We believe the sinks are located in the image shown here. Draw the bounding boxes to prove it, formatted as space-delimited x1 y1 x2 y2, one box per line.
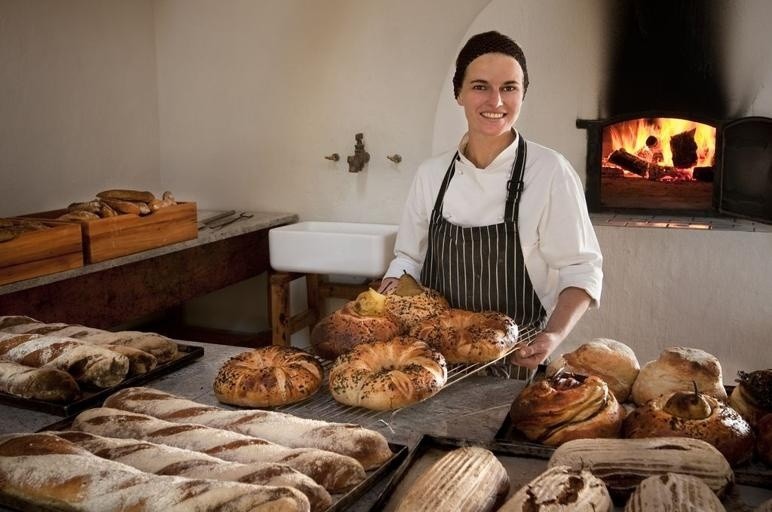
269 220 400 279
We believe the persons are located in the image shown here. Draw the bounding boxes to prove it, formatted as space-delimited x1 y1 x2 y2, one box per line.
378 31 603 382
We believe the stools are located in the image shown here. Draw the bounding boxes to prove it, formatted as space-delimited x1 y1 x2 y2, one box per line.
270 267 379 347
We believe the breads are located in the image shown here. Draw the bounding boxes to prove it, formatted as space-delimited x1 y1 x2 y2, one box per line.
409 308 518 362
547 437 735 499
0 331 129 387
533 338 640 405
510 372 627 445
71 406 366 494
329 336 447 410
624 473 726 512
0 315 178 364
632 345 727 407
620 380 754 470
0 432 311 512
101 343 157 374
0 361 80 401
730 369 772 425
103 386 392 472
396 446 510 512
48 430 333 510
310 269 450 358
497 465 614 512
213 343 324 408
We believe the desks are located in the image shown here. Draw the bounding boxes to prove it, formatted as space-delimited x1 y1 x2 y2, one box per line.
0 324 770 512
2 195 298 326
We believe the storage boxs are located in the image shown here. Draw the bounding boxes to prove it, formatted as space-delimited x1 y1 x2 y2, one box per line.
1 217 85 287
21 182 196 266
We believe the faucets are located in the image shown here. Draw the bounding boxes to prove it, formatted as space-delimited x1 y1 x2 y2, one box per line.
347 132 371 172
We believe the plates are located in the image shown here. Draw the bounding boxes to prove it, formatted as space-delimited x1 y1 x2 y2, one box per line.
1 343 206 417
370 434 771 511
36 405 410 512
494 365 771 488
